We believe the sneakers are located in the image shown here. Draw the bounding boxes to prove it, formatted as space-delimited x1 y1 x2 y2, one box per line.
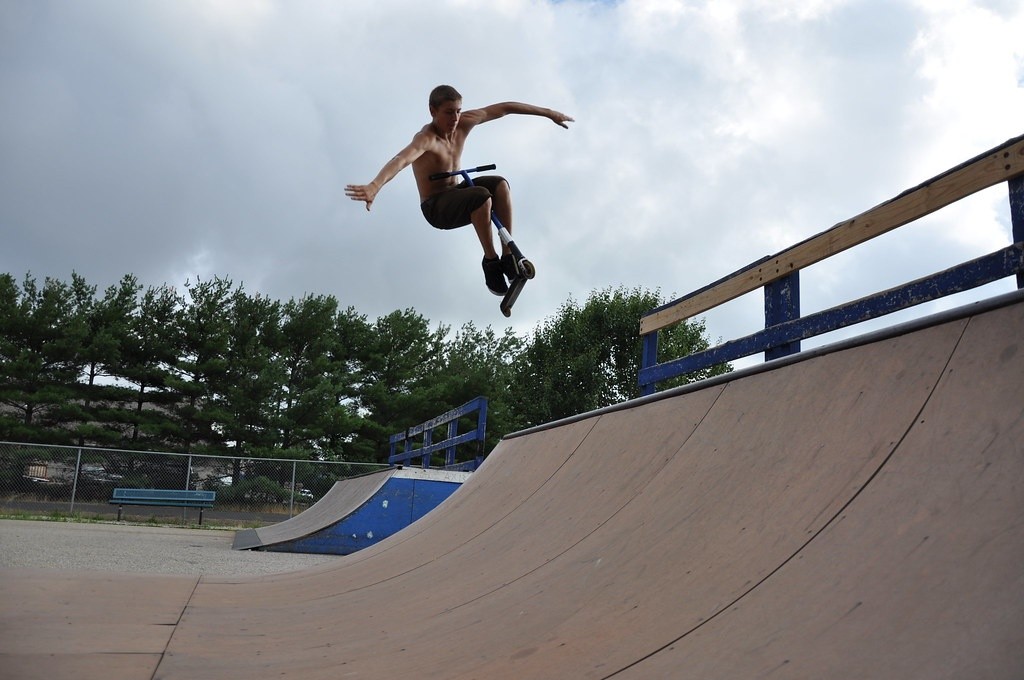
482 257 509 296
500 257 518 283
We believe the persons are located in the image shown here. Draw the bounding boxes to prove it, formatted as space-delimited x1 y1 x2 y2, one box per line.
344 85 575 296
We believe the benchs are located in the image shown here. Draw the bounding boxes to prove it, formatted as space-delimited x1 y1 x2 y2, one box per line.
109 488 216 525
